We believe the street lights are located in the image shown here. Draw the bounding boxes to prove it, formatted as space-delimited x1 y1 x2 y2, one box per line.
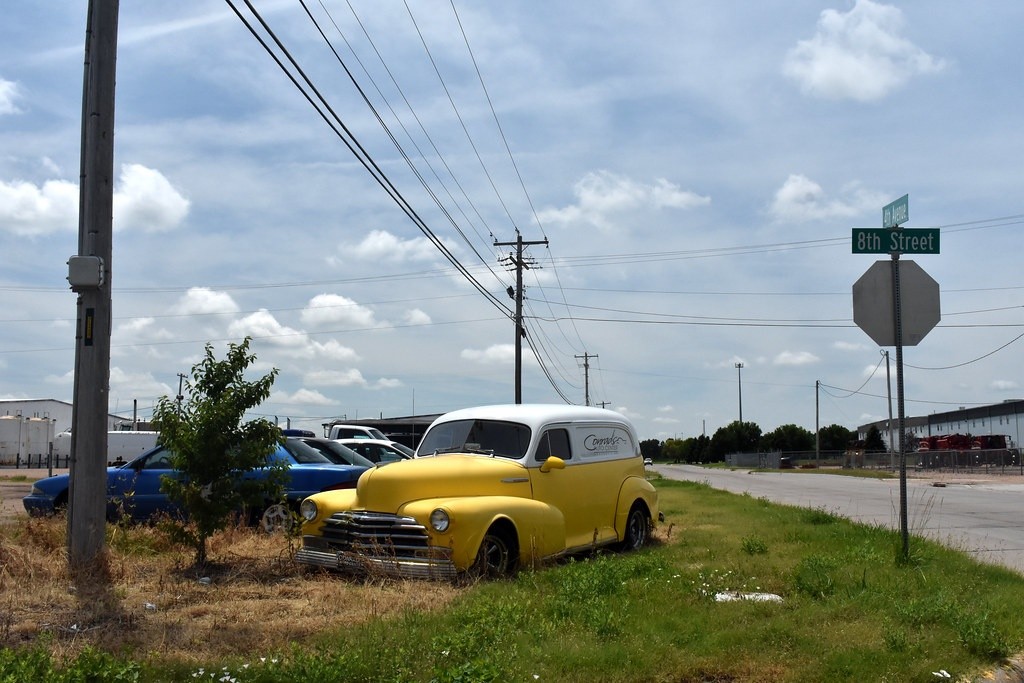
734 361 744 423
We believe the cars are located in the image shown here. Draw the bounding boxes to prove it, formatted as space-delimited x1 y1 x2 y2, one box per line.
766 455 792 468
644 458 653 466
293 404 659 585
21 425 415 537
